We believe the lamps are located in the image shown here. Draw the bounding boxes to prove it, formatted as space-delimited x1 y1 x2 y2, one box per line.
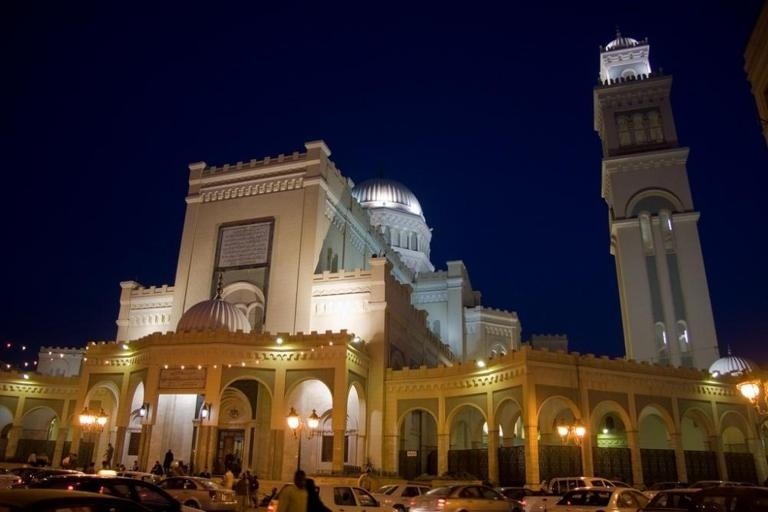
201 402 211 421
139 402 150 419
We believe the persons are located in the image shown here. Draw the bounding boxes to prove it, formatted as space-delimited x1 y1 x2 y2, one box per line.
28 450 37 467
358 468 372 491
391 503 407 512
150 449 329 511
62 442 140 473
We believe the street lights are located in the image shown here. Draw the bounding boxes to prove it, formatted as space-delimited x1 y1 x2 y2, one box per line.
139 401 151 471
201 401 213 466
735 368 768 428
555 417 588 477
78 407 109 465
285 407 321 471
